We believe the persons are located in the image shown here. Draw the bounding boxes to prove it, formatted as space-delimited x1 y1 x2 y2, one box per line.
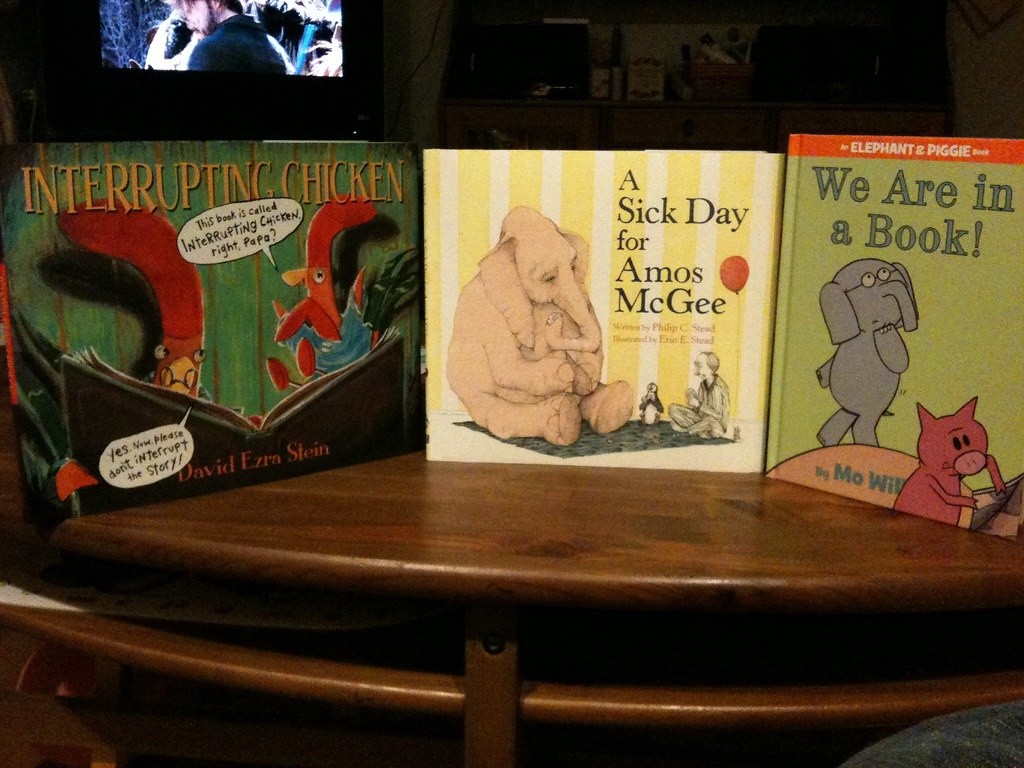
143 1 295 76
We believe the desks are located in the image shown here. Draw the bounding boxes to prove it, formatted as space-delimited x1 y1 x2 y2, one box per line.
1 378 1024 768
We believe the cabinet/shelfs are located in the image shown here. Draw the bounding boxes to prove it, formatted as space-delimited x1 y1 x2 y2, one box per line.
440 103 948 153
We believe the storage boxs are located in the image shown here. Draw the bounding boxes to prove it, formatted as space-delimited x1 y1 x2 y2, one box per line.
627 64 665 100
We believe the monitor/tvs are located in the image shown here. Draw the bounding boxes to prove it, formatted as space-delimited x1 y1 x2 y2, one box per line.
79 0 383 142
